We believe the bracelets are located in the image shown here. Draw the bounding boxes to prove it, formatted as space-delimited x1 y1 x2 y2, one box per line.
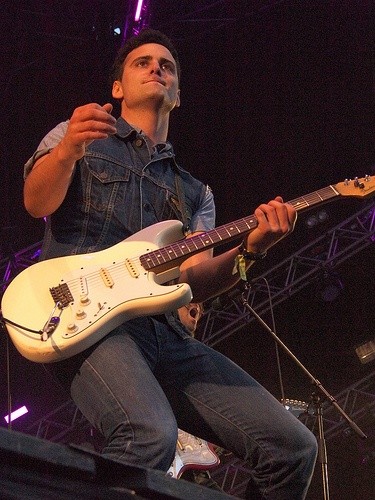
232 238 267 280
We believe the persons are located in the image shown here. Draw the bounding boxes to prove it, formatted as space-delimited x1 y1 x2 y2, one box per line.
23 28 318 500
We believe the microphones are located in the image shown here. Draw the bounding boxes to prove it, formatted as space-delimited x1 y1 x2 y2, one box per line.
210 278 256 310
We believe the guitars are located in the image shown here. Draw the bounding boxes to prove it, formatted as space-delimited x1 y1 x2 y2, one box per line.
0 176 375 363
